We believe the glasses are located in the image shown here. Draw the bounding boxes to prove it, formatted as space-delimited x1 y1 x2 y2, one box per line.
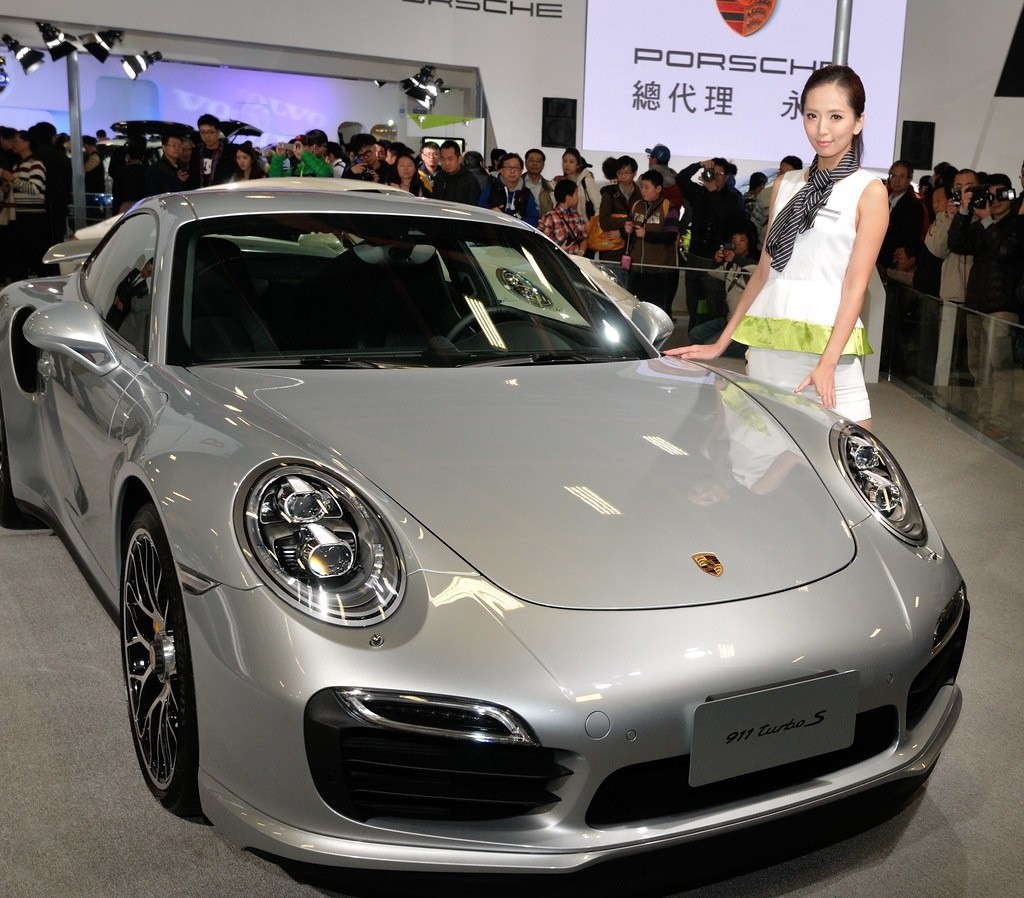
617 170 633 176
502 165 521 171
322 144 328 148
12 138 25 144
716 172 725 178
423 152 441 158
200 129 217 136
889 173 909 181
647 155 654 159
358 146 373 161
183 148 192 152
165 144 182 150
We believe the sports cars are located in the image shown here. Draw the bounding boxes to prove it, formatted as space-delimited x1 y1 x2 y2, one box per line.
1 175 970 875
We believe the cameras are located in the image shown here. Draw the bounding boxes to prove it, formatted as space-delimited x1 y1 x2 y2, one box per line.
702 168 714 182
720 243 735 251
968 184 1016 209
359 167 374 182
952 189 962 206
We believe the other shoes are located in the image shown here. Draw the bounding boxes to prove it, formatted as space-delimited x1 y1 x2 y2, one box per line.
981 424 1010 441
976 415 990 430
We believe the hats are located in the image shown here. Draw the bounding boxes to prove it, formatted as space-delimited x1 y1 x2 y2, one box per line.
934 162 951 175
295 135 311 145
579 156 592 167
645 144 670 162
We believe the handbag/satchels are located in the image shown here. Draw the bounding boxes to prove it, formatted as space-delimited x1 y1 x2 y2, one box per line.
581 177 595 221
588 188 630 251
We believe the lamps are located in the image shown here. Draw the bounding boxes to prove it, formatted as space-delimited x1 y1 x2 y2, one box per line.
79 29 124 63
0 55 9 93
402 66 451 110
120 51 163 81
36 21 78 62
2 34 45 75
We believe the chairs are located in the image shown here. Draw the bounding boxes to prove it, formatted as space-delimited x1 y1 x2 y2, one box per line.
189 238 282 354
335 238 475 348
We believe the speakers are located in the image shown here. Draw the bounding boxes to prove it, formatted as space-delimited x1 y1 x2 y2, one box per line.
900 121 936 170
542 97 577 149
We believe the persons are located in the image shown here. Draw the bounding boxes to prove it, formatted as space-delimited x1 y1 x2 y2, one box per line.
0 113 1024 441
662 65 890 433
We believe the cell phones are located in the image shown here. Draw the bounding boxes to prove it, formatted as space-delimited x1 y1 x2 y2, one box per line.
282 143 296 151
